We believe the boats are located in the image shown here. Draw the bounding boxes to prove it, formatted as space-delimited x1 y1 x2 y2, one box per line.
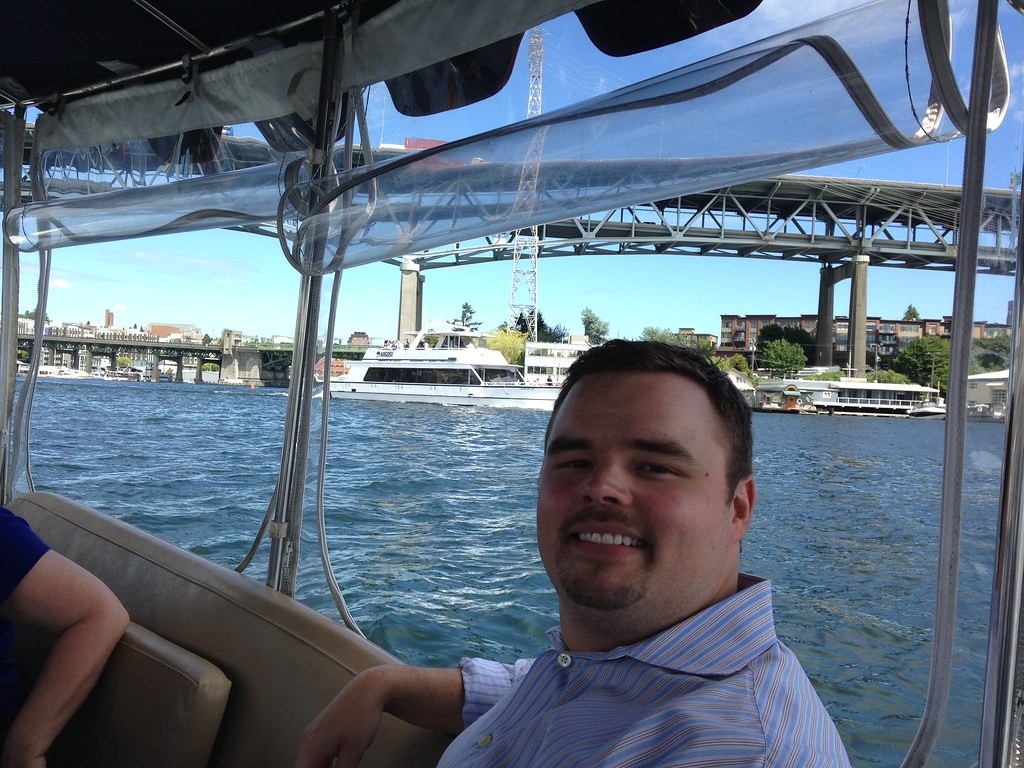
311 319 565 411
905 398 946 421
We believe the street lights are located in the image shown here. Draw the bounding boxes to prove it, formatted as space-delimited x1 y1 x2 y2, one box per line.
926 352 939 402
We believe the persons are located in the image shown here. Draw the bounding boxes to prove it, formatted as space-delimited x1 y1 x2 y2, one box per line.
0 503 130 768
294 340 849 768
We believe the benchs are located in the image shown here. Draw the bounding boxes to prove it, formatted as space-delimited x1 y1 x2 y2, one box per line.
5 492 458 768
